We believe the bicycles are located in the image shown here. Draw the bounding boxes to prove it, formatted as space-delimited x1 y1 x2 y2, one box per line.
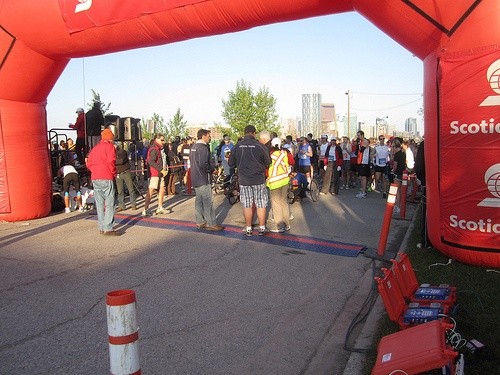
212 163 320 206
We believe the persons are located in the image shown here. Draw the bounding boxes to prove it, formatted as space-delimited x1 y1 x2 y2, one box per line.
228 126 272 236
165 136 195 194
264 138 290 232
128 141 148 183
58 165 88 213
69 108 88 166
86 101 106 151
86 128 121 236
258 130 426 221
113 142 138 211
190 129 225 232
142 133 171 217
52 137 78 165
216 134 243 183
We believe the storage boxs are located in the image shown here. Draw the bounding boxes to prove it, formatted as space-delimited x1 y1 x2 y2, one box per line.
374 267 451 329
390 250 460 304
371 320 458 375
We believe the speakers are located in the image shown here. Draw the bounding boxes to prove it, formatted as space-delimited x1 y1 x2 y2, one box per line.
104 115 143 160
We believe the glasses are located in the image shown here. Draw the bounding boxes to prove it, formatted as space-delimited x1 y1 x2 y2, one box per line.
158 139 164 141
380 139 384 140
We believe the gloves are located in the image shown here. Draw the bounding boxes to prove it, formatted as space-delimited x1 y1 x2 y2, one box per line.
324 166 327 171
336 166 341 171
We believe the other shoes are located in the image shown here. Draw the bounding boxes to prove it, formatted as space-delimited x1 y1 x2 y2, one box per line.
269 228 284 232
116 207 125 212
258 229 265 235
142 211 156 216
132 206 136 210
246 227 252 237
290 215 294 220
206 223 225 231
285 225 290 231
103 230 121 236
64 208 70 213
156 208 171 214
319 187 385 198
196 221 206 228
79 207 84 211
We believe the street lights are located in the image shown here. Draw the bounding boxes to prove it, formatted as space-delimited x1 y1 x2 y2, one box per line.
346 88 351 140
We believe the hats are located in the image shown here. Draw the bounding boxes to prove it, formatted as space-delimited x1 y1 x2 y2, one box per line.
330 137 336 140
76 107 84 113
225 136 230 140
102 129 114 140
321 135 328 139
272 138 281 147
